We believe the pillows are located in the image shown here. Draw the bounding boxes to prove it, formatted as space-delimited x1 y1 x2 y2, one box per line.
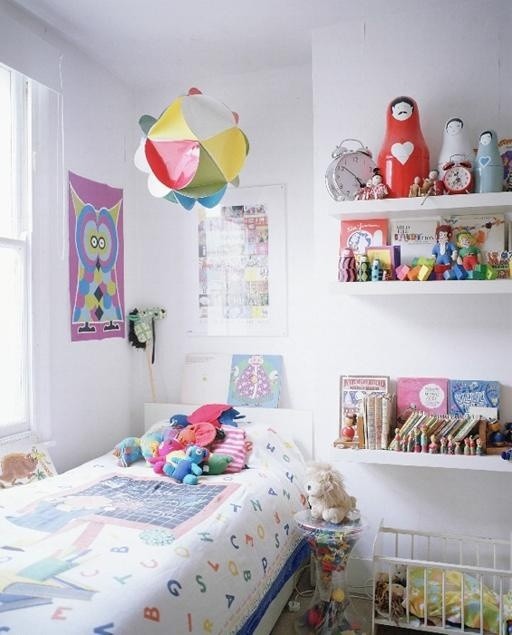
401 562 506 634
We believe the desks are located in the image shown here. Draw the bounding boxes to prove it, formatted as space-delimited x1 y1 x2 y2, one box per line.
294 512 369 635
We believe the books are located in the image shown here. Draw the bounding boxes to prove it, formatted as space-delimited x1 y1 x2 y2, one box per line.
338 373 501 455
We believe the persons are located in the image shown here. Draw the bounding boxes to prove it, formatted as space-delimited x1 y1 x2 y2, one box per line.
377 580 443 625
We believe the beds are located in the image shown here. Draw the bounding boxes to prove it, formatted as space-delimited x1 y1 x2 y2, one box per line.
1 401 315 634
369 519 512 635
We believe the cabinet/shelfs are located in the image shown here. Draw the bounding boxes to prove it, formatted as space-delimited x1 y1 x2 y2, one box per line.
332 192 512 477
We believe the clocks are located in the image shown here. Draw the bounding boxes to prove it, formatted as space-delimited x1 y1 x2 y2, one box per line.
441 152 473 195
323 137 380 201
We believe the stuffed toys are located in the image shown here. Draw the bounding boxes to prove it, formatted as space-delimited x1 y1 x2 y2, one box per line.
112 403 253 486
303 461 360 523
376 573 405 609
